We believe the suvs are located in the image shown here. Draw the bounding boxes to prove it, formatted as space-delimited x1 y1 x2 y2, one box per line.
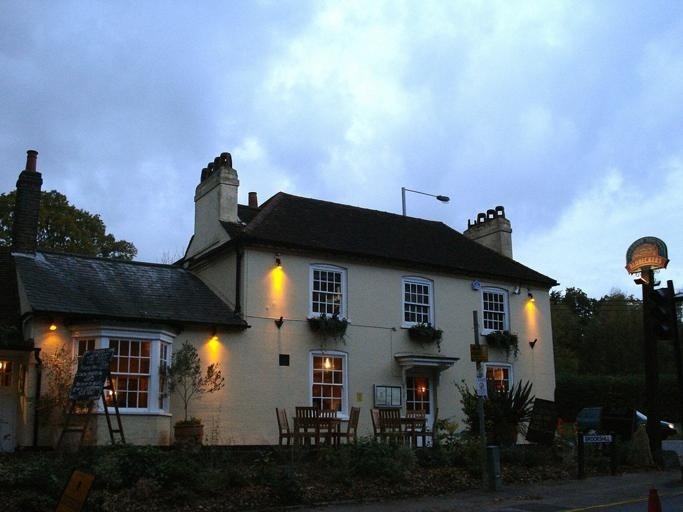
577 405 677 441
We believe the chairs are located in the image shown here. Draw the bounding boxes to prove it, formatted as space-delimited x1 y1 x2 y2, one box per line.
275 405 360 447
370 406 439 449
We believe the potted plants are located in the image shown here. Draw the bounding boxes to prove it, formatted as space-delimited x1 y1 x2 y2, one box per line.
157 338 225 444
452 376 541 449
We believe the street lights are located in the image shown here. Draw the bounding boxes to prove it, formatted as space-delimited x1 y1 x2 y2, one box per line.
400 187 449 219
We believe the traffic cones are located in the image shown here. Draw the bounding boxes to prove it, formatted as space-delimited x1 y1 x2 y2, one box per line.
646 486 664 512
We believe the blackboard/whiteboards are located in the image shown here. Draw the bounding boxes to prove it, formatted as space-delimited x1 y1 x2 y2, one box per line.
69 347 115 401
526 399 558 443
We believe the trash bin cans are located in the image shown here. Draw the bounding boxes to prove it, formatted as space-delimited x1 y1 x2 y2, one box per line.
576 405 634 478
